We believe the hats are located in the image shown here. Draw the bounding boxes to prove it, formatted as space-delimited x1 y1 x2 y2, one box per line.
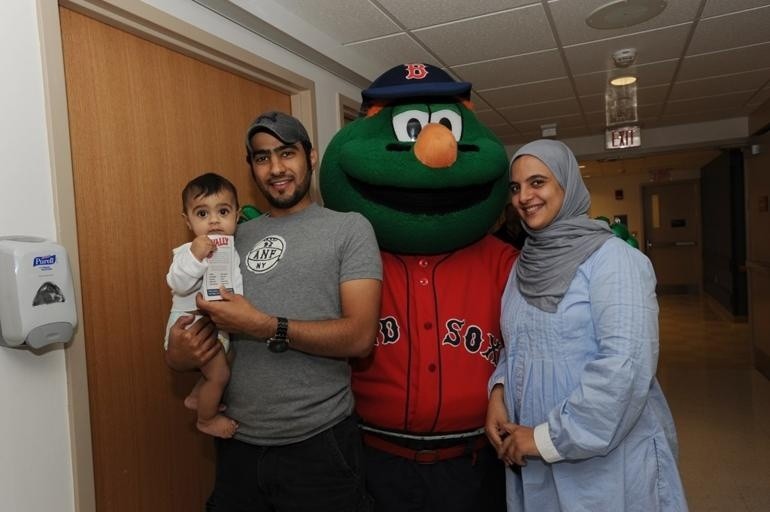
245 111 310 158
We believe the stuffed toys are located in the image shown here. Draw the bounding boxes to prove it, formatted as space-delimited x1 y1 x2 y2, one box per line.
320 63 522 512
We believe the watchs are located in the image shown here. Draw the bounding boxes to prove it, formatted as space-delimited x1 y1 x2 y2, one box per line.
266 317 290 353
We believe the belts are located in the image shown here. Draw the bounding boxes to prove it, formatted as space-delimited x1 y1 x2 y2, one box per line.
359 429 491 466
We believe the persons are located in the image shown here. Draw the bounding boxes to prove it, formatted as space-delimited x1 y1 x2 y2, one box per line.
164 111 383 512
486 138 689 512
161 173 244 439
